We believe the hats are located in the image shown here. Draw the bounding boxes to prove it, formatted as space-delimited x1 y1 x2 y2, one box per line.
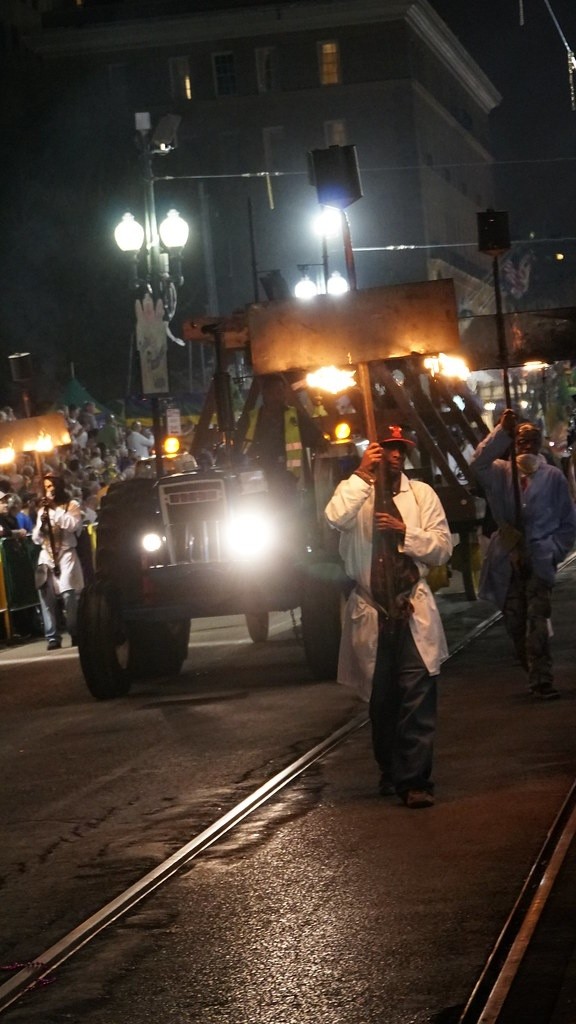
0 491 12 500
42 472 63 479
377 425 417 448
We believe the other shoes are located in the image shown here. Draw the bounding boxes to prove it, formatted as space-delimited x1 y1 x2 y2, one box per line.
530 685 560 699
47 642 61 650
378 774 395 795
406 790 435 807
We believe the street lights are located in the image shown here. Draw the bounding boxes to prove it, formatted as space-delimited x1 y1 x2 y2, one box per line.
294 207 349 300
114 209 189 478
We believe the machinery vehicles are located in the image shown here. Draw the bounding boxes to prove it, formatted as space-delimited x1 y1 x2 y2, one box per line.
73 111 493 701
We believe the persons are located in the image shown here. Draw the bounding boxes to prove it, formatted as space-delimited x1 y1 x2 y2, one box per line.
469 408 576 702
233 376 329 514
324 422 452 807
0 400 226 650
